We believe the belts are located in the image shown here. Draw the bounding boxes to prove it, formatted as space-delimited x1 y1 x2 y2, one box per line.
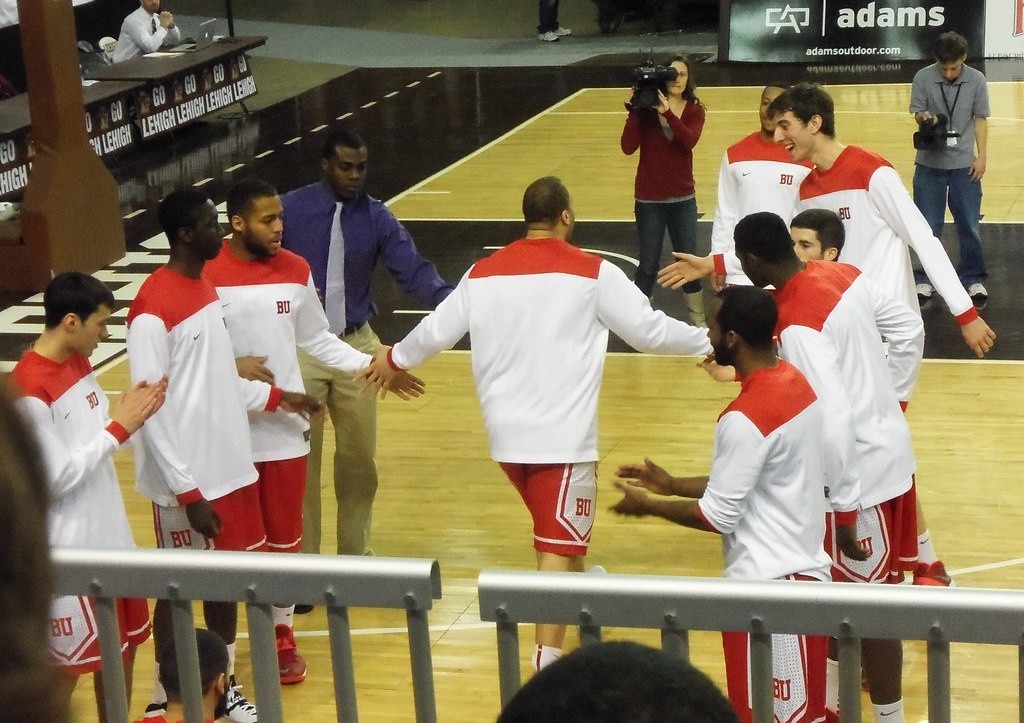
340 320 366 337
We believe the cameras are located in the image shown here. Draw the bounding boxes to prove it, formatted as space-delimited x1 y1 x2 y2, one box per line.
913 113 961 150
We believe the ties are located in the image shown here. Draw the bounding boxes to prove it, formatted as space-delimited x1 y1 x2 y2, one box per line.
151 18 157 34
326 201 346 337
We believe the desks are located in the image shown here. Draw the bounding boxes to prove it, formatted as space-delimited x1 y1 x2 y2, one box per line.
0 80 145 203
84 36 269 150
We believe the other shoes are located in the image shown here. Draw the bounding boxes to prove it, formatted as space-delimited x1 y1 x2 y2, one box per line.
292 603 314 613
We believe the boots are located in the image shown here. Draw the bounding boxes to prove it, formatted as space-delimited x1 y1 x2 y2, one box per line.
684 288 706 328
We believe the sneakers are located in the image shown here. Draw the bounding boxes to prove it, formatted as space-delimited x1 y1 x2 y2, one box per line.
274 623 307 684
553 26 572 36
538 31 560 42
915 283 933 301
223 681 257 723
968 282 988 310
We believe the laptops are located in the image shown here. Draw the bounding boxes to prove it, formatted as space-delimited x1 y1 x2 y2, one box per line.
168 18 216 52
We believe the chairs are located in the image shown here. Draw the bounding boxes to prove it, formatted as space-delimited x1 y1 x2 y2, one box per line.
99 36 117 59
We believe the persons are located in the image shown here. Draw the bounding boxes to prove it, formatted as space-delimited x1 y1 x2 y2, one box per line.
8 271 170 723
132 628 231 723
621 56 709 330
279 129 456 615
201 178 425 685
352 175 714 675
606 81 1024 723
497 640 740 723
909 32 991 300
112 0 182 65
126 186 329 723
538 0 572 42
